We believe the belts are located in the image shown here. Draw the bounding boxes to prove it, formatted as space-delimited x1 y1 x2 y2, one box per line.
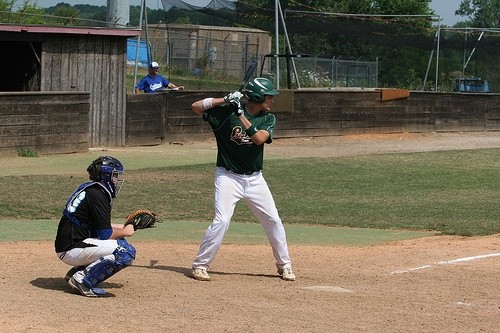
226 168 254 175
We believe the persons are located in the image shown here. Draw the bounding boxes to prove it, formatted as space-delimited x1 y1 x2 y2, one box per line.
135 61 178 94
54 156 136 296
191 77 297 281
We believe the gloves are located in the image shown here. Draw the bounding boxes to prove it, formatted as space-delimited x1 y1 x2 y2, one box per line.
224 91 244 103
230 99 243 117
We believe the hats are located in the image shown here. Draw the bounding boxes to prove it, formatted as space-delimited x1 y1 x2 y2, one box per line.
149 62 159 69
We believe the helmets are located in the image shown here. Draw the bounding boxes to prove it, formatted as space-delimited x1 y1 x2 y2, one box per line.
244 78 280 103
86 156 125 198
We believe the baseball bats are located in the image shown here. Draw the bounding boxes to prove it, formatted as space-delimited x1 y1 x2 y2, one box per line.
230 59 259 109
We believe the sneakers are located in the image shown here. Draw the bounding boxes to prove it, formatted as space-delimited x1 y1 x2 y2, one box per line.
68 276 98 296
65 267 106 294
191 268 210 281
278 267 295 281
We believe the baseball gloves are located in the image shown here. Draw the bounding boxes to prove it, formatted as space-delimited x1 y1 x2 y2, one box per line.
123 210 158 231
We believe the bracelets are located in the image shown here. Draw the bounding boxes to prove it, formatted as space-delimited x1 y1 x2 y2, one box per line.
245 124 258 136
